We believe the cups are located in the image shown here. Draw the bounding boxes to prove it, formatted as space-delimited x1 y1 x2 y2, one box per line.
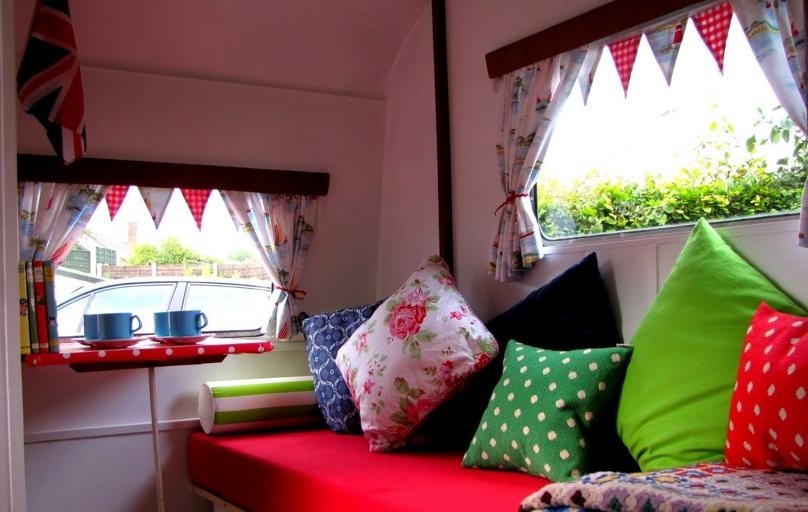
83 313 141 339
152 310 207 335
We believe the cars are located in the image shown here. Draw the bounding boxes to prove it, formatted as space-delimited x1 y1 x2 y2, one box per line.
53 266 288 343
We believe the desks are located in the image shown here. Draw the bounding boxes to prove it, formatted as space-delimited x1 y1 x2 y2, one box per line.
23 336 274 512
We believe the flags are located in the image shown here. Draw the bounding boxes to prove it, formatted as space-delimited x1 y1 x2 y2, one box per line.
15 0 89 167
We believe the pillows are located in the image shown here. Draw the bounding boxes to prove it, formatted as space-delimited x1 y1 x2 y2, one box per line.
301 297 390 433
724 302 808 471
334 255 501 452
197 376 327 435
425 253 618 454
460 338 633 482
603 216 808 472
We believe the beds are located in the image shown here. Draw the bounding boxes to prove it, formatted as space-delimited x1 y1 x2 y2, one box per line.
188 426 555 512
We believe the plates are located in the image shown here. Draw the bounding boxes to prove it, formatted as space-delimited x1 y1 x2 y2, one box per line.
71 338 146 348
147 336 215 343
